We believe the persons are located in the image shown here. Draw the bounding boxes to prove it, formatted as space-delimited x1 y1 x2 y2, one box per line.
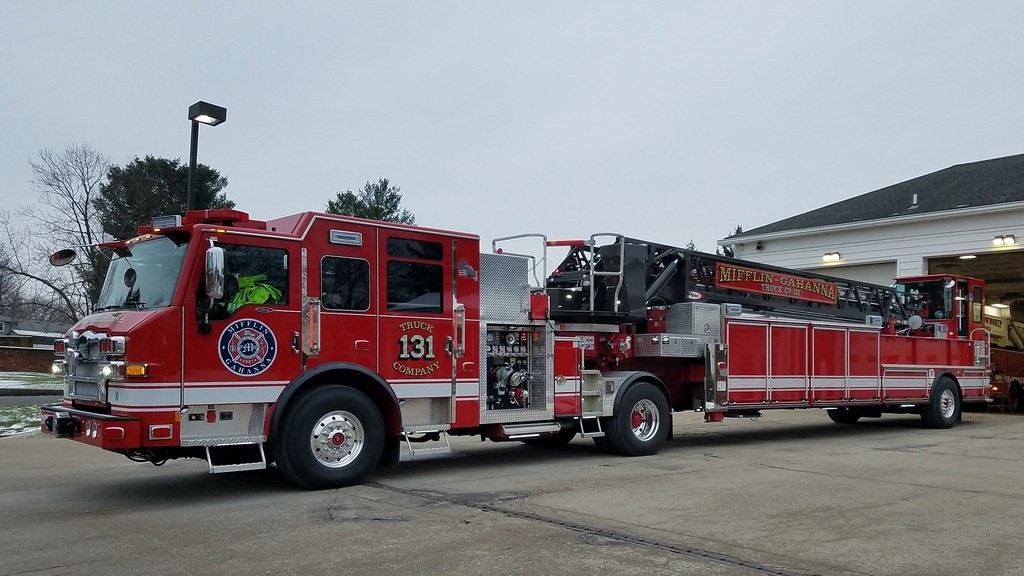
221 260 283 316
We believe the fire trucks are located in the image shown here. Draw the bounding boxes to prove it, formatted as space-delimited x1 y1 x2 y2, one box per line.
39 208 1024 492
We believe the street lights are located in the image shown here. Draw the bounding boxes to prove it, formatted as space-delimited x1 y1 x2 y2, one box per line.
185 100 227 213
